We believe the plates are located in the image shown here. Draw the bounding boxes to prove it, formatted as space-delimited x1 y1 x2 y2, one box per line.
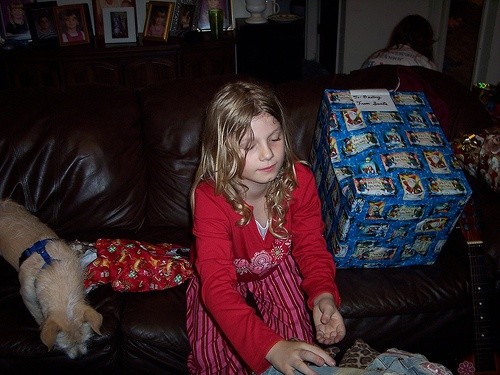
268 14 304 24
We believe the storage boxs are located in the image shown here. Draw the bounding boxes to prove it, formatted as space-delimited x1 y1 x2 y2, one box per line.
308 88 472 270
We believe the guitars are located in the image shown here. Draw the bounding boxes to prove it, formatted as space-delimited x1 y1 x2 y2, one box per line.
454 201 500 375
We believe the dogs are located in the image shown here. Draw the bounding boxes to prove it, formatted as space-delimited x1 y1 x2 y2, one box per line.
0 199 103 360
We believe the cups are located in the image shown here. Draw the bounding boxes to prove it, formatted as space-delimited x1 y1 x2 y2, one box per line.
208 8 224 40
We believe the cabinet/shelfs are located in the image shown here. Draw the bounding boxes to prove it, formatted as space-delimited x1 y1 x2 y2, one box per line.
0 30 235 98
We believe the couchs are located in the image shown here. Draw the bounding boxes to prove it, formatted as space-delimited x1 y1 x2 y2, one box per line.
0 75 500 375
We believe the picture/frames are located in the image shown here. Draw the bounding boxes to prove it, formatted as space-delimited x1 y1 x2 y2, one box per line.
24 1 57 44
142 0 175 43
102 7 137 43
0 0 30 38
170 0 203 37
53 5 90 47
92 0 138 40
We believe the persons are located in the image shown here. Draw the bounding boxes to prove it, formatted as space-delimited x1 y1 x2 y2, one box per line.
185 82 347 375
360 14 438 71
182 8 191 30
148 10 165 37
62 12 86 42
36 13 57 39
5 2 27 34
